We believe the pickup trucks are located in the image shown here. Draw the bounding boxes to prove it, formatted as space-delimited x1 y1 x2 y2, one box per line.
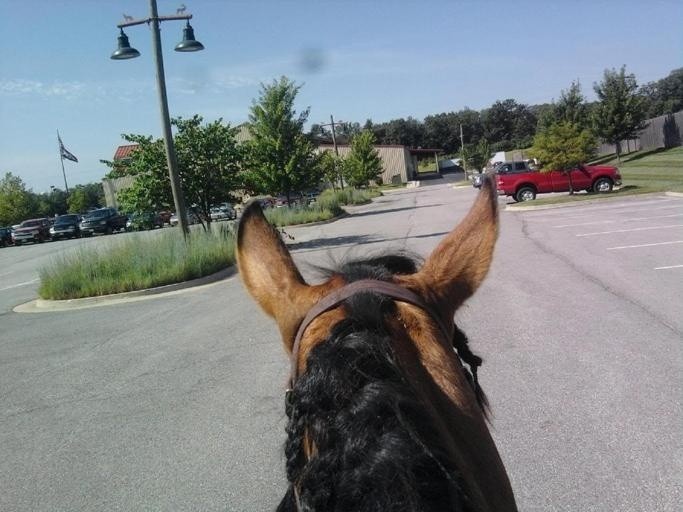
496 158 624 205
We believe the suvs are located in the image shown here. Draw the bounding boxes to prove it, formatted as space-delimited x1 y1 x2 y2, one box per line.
472 159 533 194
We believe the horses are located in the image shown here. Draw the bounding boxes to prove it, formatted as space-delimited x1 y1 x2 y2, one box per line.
234 171 518 511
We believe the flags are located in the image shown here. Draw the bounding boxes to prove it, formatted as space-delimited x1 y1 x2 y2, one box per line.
57 136 77 162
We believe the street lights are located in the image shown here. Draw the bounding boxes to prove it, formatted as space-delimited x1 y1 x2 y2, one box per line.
449 122 469 183
52 189 55 211
319 114 353 195
108 1 206 250
48 185 53 209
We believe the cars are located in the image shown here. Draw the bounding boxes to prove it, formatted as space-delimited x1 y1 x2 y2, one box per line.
0 194 298 251
